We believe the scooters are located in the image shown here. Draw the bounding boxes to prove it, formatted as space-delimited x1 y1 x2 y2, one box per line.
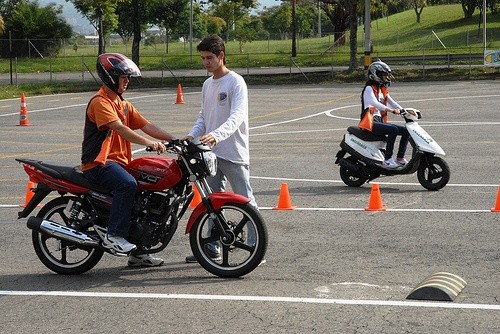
333 106 451 193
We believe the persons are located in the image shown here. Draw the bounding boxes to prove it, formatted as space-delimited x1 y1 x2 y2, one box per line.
357 61 412 169
79 52 181 266
180 33 265 267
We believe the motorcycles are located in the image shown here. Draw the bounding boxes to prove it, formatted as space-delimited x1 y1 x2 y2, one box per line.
13 134 271 280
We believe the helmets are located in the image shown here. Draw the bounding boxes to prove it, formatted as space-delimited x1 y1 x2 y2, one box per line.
368 61 392 87
96 52 134 92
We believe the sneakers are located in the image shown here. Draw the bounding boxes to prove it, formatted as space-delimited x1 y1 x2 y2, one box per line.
127 251 164 267
102 232 137 254
384 158 400 169
397 158 409 166
186 250 221 263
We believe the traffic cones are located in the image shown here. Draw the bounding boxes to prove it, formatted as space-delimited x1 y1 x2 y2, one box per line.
363 182 386 212
17 92 32 127
18 177 45 208
271 182 298 210
187 180 203 209
173 83 185 104
489 183 500 214
